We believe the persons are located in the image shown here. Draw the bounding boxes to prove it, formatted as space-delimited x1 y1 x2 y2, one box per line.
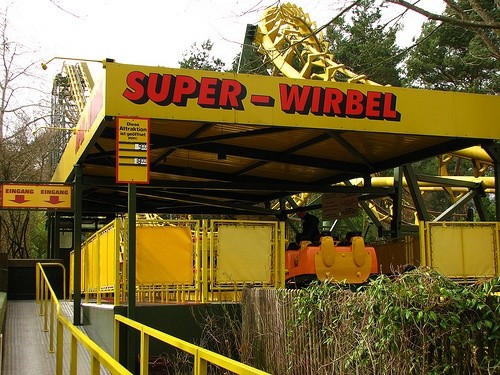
295 206 320 243
